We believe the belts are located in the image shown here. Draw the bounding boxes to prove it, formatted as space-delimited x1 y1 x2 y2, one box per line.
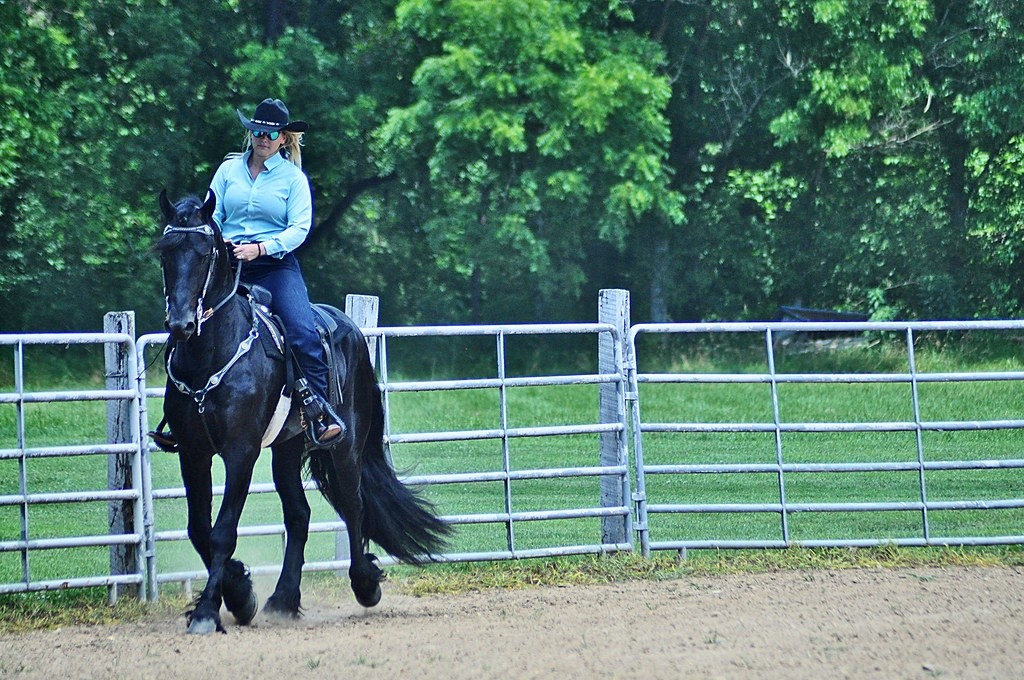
233 240 262 244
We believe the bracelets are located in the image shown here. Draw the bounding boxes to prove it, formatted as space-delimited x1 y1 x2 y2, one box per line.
258 243 261 257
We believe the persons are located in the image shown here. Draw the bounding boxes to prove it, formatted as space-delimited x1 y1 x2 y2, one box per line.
146 97 342 454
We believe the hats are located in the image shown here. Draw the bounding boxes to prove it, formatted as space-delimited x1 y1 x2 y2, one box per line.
236 97 309 135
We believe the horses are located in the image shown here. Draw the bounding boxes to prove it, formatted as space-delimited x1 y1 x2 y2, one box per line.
151 186 459 635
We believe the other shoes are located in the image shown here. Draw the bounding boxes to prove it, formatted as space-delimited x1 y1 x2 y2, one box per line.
312 417 342 441
147 430 176 446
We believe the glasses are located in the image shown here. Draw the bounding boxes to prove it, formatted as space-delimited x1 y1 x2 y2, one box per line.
251 130 283 141
